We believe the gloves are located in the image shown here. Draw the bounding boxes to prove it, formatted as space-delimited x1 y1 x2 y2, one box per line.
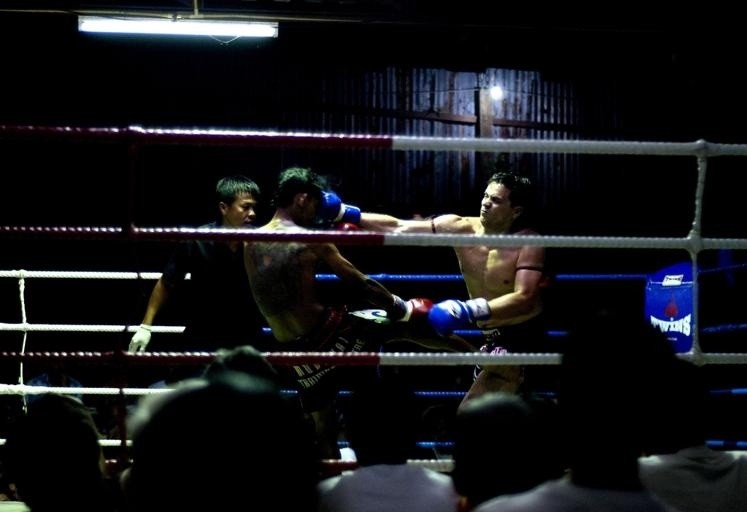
386 293 433 339
428 297 492 339
128 323 152 357
314 191 361 225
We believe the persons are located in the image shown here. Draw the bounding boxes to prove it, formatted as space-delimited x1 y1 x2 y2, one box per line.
0 312 747 512
126 173 276 382
238 159 495 458
315 169 571 407
